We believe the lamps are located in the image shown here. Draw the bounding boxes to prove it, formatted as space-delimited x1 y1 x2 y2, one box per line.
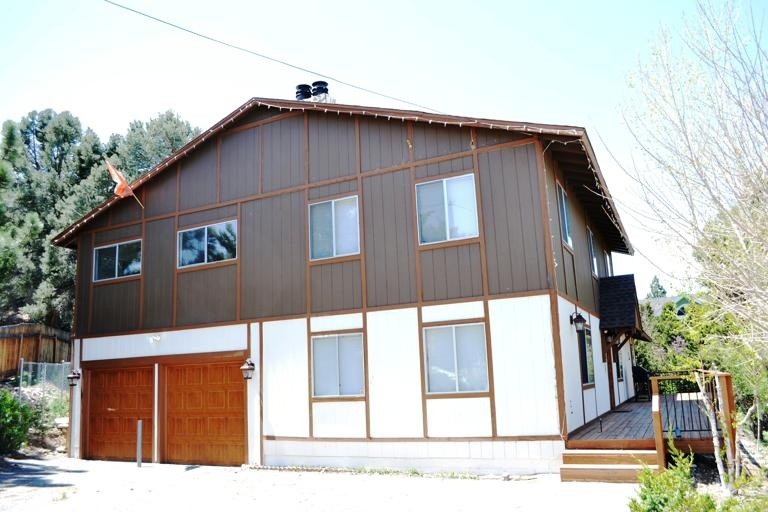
67 369 80 386
240 358 255 380
570 312 586 331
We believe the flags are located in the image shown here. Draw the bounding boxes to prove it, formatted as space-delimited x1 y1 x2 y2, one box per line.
104 157 133 199
